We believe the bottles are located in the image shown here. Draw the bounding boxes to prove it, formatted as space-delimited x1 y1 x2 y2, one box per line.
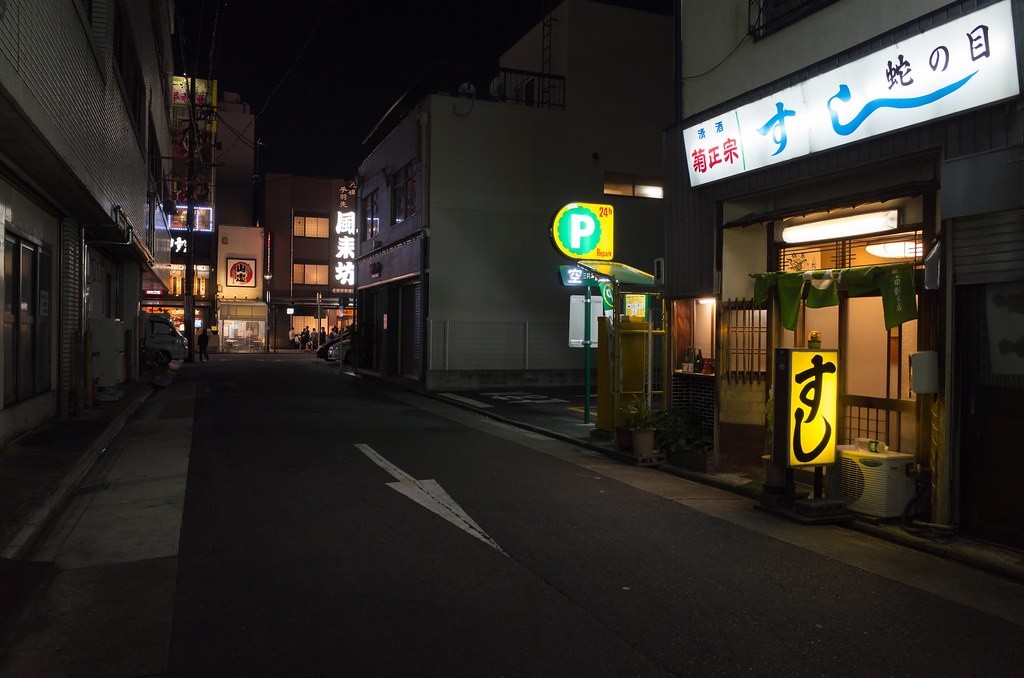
681 346 704 373
854 438 890 454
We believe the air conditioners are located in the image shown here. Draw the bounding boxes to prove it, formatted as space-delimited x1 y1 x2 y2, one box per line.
826 445 917 518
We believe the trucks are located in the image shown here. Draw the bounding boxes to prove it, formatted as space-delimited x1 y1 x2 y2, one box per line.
145 314 189 363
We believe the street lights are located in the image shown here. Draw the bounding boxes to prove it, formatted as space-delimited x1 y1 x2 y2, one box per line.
264 273 273 352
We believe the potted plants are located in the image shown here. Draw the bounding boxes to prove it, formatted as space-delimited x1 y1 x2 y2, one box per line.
615 394 655 454
655 409 715 473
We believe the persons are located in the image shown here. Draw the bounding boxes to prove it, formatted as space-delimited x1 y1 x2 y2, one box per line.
196 329 210 361
300 323 355 353
287 326 299 349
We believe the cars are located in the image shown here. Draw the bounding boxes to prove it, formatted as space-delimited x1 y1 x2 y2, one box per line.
316 330 351 362
328 340 354 365
